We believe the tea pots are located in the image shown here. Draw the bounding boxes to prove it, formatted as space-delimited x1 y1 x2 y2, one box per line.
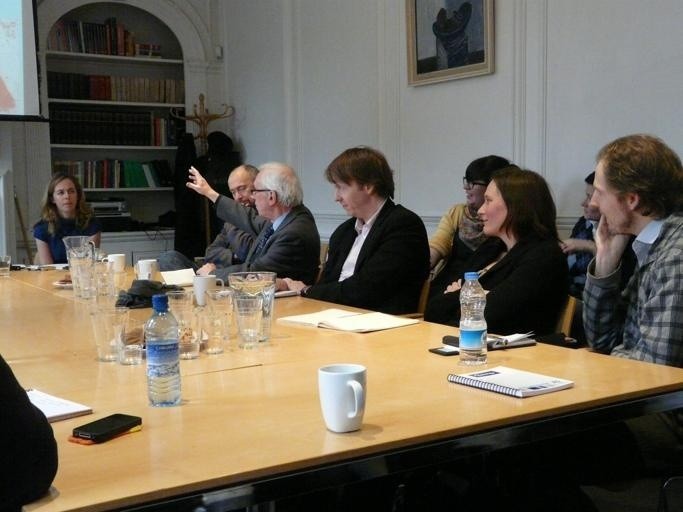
61 233 101 300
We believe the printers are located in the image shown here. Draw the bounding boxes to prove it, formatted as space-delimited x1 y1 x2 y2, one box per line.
86 197 131 217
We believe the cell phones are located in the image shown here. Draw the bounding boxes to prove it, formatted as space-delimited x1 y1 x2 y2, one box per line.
73 414 142 442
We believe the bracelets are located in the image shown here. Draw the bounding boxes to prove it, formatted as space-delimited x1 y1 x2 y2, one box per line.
299 285 312 295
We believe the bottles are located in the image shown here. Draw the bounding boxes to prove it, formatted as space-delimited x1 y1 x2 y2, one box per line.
144 294 182 407
459 271 489 366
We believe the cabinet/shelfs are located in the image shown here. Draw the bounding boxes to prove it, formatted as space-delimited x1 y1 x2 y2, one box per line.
43 43 187 194
90 229 177 267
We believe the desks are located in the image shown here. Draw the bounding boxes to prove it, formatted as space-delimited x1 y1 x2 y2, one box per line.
1 323 682 512
5 268 429 366
3 276 209 360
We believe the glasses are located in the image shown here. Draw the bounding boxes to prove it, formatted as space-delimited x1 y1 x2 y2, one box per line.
250 190 270 199
462 176 485 189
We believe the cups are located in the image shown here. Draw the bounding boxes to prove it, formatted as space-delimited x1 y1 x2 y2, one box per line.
100 252 127 275
202 289 232 322
168 304 198 359
228 270 275 350
166 290 193 311
110 315 143 367
132 259 160 281
0 255 12 279
192 275 223 307
87 306 128 363
198 308 227 353
319 363 368 435
234 294 263 350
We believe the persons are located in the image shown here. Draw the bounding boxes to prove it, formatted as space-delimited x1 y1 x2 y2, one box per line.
557 171 608 344
1 353 59 512
423 164 567 343
34 171 102 266
183 162 321 291
159 165 268 275
496 132 683 511
426 154 513 273
262 148 430 315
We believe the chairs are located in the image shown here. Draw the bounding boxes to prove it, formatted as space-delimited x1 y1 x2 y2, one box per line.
317 243 578 341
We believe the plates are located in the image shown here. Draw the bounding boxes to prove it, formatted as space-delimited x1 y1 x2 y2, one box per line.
52 280 73 291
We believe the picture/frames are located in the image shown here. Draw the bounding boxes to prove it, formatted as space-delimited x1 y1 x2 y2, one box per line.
406 0 497 90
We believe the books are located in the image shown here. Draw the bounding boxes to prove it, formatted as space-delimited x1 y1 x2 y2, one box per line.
25 389 92 423
277 308 419 333
448 366 574 399
46 17 187 219
428 330 536 356
160 268 198 287
273 290 298 299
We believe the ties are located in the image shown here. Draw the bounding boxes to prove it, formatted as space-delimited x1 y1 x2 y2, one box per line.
255 223 273 255
